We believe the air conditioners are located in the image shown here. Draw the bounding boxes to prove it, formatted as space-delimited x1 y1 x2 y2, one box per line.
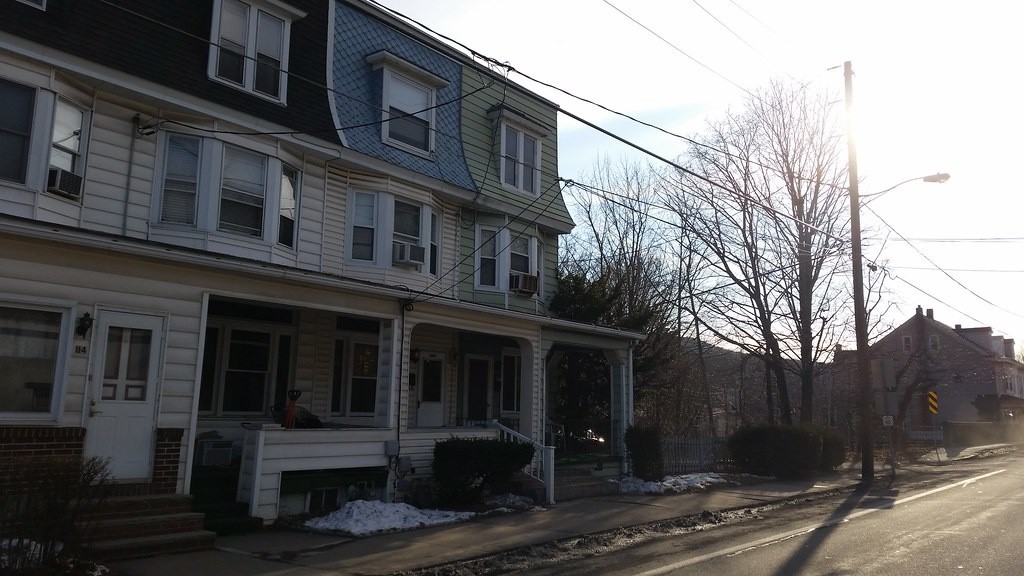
194 439 232 467
510 273 538 293
395 243 426 264
48 167 84 198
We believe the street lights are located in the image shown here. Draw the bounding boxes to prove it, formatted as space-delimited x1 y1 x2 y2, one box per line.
849 173 952 480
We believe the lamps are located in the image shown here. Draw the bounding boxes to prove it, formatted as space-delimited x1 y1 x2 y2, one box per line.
409 348 423 363
77 312 94 335
